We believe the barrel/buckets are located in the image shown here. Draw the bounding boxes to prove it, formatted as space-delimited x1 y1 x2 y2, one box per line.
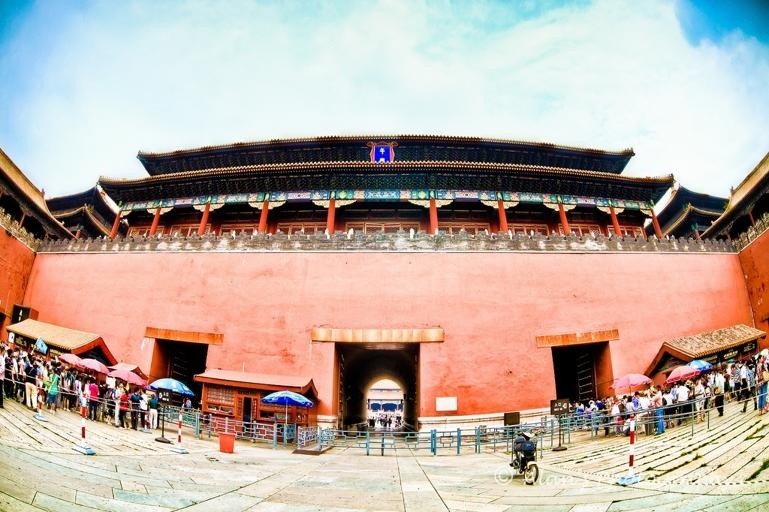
219 432 235 453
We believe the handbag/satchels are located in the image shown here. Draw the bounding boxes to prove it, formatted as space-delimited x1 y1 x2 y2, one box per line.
761 370 769 381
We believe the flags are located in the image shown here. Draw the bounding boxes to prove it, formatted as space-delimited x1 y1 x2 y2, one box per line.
36 337 48 355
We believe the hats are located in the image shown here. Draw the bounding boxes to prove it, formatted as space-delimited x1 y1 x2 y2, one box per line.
522 430 536 438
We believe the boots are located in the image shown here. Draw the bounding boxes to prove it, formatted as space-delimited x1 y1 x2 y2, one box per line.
754 401 758 411
740 406 747 413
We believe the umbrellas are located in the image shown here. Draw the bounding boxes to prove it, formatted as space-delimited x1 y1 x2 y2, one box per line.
148 374 195 397
262 389 314 424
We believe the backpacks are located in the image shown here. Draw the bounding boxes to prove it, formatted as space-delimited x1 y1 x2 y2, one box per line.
524 440 535 451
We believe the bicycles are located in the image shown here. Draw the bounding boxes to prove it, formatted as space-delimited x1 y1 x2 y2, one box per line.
510 427 543 484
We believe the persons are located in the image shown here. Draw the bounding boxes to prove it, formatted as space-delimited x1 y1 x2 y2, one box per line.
0 341 161 432
516 432 536 474
566 348 769 436
510 429 539 466
186 398 191 408
369 414 393 427
